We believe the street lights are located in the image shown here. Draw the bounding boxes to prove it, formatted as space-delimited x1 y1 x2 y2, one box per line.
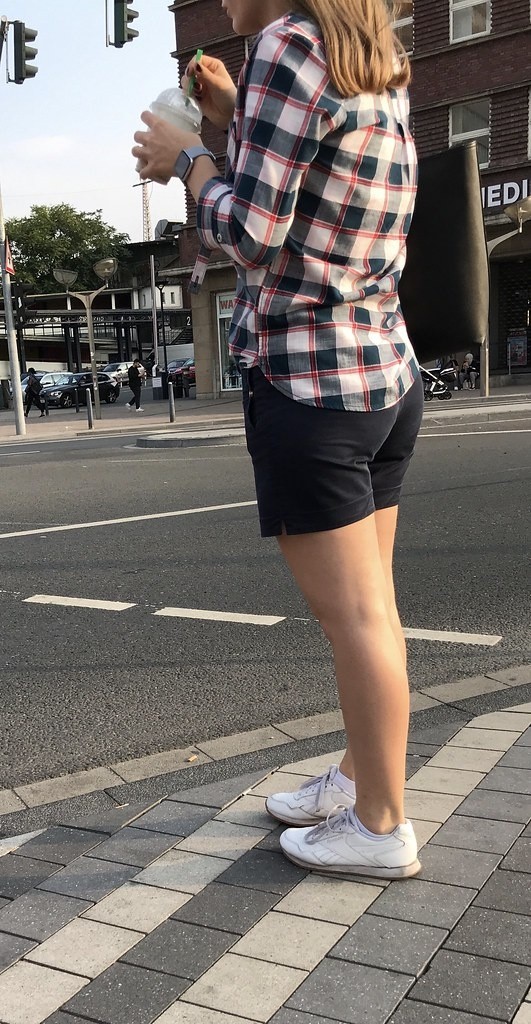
479 196 531 397
52 257 119 421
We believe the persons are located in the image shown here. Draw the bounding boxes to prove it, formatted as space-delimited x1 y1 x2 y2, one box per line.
459 353 480 391
438 354 459 390
125 358 145 412
131 0 424 880
24 368 45 418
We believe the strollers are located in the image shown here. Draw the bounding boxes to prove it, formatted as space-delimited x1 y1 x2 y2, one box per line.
418 365 452 400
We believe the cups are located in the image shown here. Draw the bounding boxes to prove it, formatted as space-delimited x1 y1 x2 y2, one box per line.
136 86 203 185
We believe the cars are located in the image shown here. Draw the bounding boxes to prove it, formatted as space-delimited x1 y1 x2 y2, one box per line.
159 358 189 375
173 359 196 384
40 373 119 408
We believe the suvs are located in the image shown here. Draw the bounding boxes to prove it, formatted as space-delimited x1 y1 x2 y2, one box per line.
9 373 76 405
103 363 146 386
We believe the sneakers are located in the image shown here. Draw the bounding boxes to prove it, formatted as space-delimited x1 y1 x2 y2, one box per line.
265 764 357 826
280 803 421 878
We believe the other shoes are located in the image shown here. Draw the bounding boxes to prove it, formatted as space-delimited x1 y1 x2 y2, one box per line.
454 387 458 390
24 413 27 417
136 407 144 412
469 388 475 390
126 403 131 410
40 414 45 417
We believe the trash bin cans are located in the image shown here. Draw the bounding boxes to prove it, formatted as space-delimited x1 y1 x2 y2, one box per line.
152 376 169 400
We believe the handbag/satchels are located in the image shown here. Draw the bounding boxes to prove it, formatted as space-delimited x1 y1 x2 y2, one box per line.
30 375 42 394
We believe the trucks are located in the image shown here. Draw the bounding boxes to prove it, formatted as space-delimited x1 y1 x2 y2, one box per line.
141 343 194 377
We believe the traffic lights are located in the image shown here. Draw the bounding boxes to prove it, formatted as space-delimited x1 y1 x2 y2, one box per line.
6 22 38 85
14 283 38 325
106 0 139 46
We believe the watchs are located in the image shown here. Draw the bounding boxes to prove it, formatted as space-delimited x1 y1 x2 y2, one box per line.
174 147 217 187
